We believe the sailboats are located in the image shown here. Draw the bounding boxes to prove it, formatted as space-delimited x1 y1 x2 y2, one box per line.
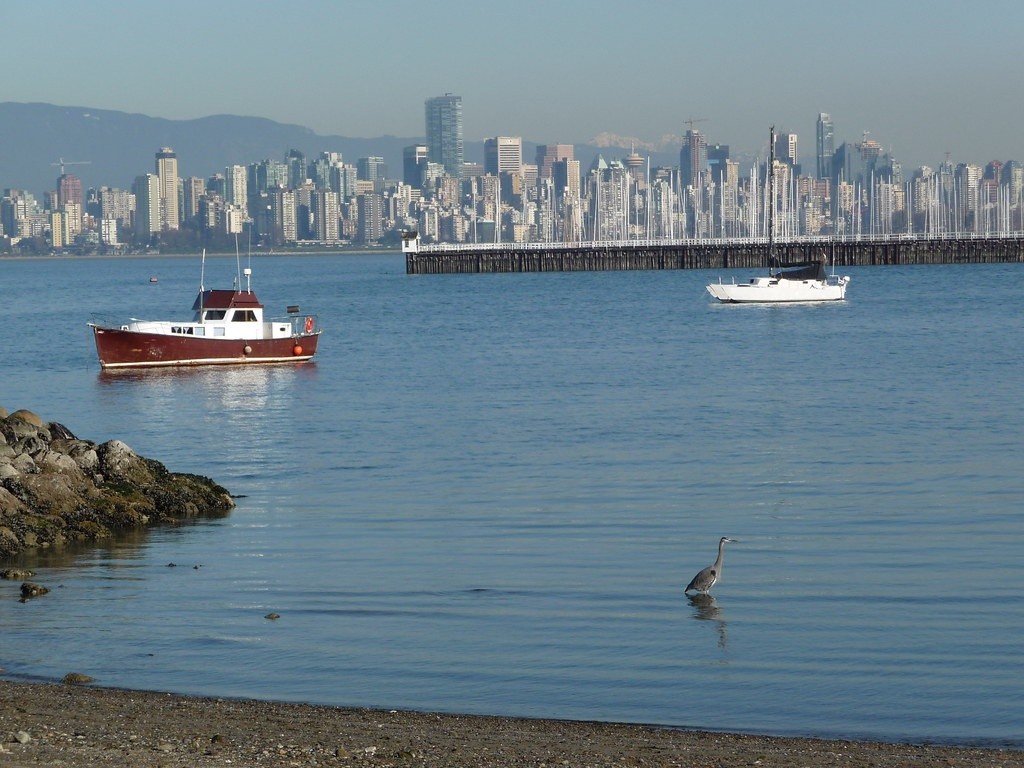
705 123 850 303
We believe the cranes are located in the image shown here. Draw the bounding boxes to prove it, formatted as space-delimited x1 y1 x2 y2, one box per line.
51 158 91 175
683 118 714 136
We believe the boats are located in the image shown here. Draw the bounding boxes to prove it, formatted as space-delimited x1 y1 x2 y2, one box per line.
86 225 323 369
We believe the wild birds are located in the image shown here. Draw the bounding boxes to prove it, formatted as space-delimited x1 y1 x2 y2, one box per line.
684 536 739 598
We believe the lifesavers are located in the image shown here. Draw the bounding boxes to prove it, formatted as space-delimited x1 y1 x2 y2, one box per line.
305 317 314 333
